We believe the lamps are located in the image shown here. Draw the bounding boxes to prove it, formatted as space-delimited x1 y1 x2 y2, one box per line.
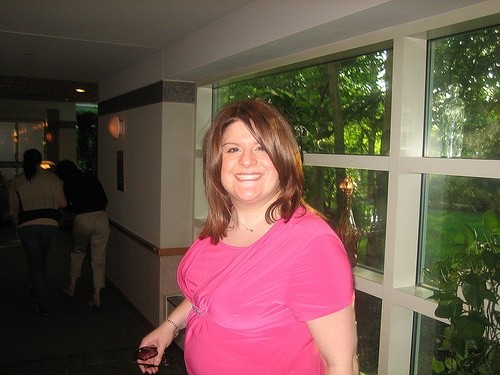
108 113 125 140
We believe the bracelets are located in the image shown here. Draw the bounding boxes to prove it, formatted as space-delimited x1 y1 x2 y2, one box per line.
164 317 179 336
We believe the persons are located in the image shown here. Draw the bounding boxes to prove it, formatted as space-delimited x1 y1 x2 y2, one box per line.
137 99 362 374
7 148 69 321
53 159 112 309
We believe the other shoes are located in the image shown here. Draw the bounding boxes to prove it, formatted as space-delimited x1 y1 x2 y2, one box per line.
86 300 102 308
63 292 74 298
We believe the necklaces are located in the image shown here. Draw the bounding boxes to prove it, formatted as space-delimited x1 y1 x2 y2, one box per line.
230 209 270 233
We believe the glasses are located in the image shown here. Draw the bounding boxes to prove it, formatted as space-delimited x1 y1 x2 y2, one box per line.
134 346 170 368
